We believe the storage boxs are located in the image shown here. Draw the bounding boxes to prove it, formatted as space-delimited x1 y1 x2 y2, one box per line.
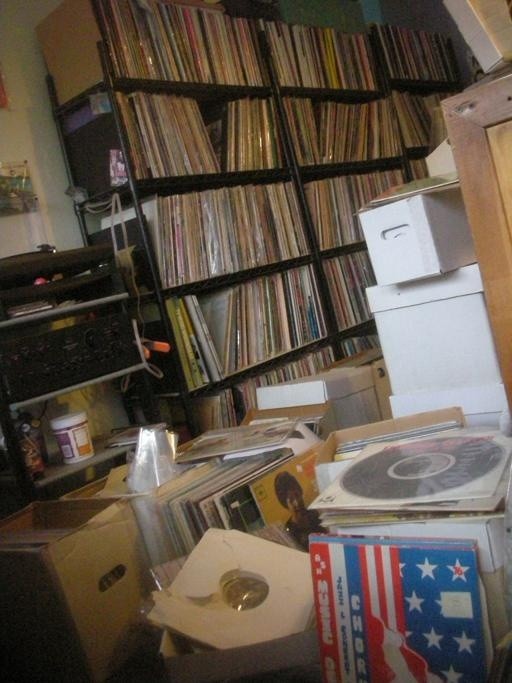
59 459 218 562
158 628 321 683
338 521 512 646
314 406 467 497
0 495 153 683
365 264 505 394
388 393 506 425
240 402 337 438
255 367 382 429
316 349 393 419
358 189 477 287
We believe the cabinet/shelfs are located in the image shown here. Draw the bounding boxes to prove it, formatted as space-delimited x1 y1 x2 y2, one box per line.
261 28 408 361
46 40 341 438
371 23 462 184
0 291 164 488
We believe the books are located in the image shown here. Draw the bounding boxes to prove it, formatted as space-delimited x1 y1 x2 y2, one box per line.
283 98 402 167
100 182 308 290
257 18 376 88
406 158 427 180
342 334 380 358
333 419 460 459
62 96 284 179
193 346 338 427
35 0 263 107
303 169 405 251
140 264 327 390
319 251 378 332
157 417 324 558
392 91 458 147
376 22 458 82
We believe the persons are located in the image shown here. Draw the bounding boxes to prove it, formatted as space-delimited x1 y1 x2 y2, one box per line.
116 151 125 171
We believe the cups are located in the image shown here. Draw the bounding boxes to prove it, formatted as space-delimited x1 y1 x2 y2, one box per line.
15 430 45 480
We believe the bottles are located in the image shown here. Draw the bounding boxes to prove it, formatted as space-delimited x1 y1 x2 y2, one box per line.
52 411 94 465
